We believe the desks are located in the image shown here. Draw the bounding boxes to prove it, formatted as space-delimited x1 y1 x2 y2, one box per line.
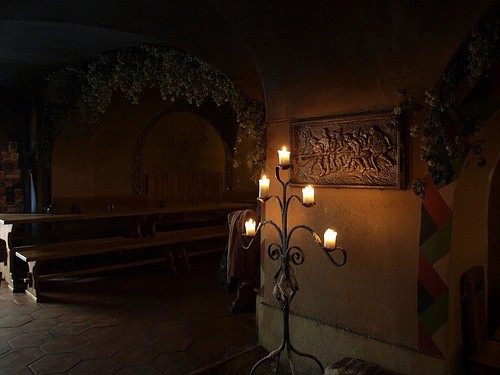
150 201 255 237
0 204 155 248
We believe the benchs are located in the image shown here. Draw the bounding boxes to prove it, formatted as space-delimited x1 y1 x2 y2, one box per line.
13 230 184 303
176 225 229 270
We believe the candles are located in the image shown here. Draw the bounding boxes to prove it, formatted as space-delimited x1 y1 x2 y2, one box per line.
324 228 337 249
278 145 291 166
257 175 271 199
302 184 314 204
244 219 257 236
